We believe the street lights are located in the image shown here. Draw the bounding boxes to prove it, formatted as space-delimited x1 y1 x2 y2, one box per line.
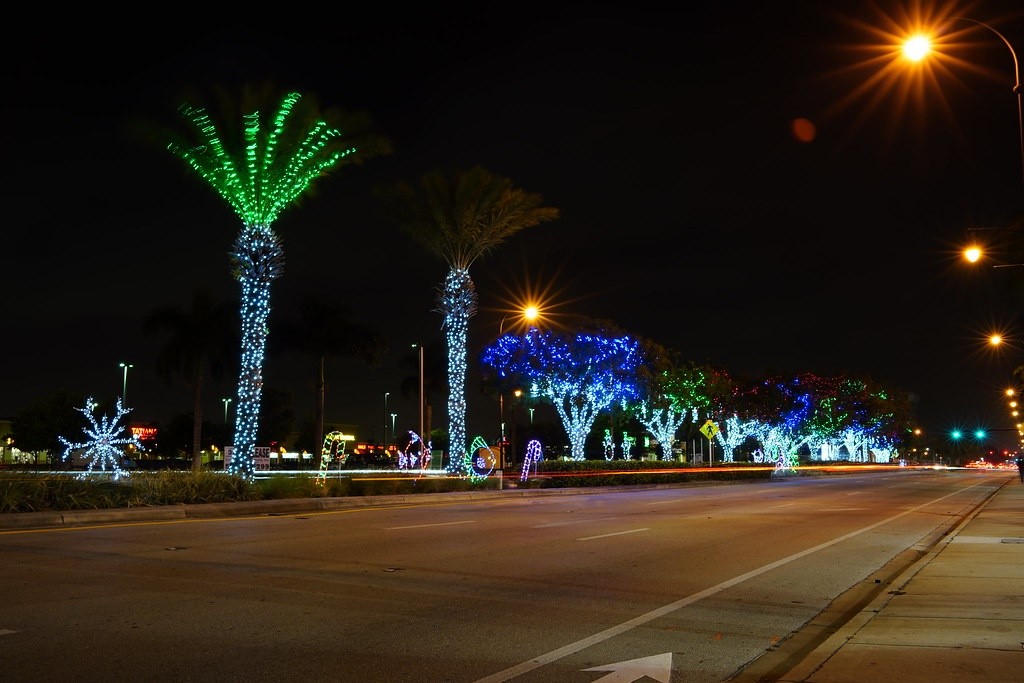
384 393 390 411
222 399 231 427
497 300 540 469
120 362 133 410
412 343 424 459
390 413 397 439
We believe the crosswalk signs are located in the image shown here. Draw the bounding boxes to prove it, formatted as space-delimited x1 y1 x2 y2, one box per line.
699 420 718 439
